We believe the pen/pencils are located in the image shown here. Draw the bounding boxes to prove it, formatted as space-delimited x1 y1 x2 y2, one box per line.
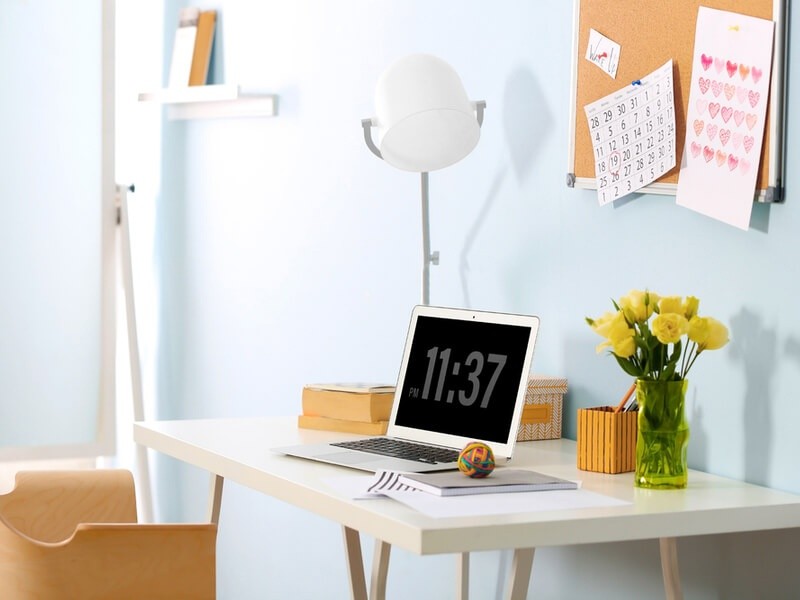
614 382 637 413
624 396 637 413
631 404 638 411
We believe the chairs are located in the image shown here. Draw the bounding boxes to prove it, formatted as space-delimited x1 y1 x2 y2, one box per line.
0 467 217 600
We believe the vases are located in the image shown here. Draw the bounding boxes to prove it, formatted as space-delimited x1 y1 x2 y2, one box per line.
637 379 689 491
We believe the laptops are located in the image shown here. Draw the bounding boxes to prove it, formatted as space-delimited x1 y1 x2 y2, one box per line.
278 306 540 473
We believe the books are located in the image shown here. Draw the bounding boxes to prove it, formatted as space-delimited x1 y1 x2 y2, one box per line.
303 385 396 394
170 8 217 87
397 466 579 497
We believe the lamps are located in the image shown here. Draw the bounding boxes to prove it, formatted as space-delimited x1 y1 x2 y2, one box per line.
359 54 486 306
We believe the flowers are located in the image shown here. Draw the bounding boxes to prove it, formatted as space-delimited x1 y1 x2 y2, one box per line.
583 289 729 476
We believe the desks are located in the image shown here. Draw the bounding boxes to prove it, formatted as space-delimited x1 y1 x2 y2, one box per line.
133 418 800 600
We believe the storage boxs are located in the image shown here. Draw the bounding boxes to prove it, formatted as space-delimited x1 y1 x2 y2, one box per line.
516 376 568 442
